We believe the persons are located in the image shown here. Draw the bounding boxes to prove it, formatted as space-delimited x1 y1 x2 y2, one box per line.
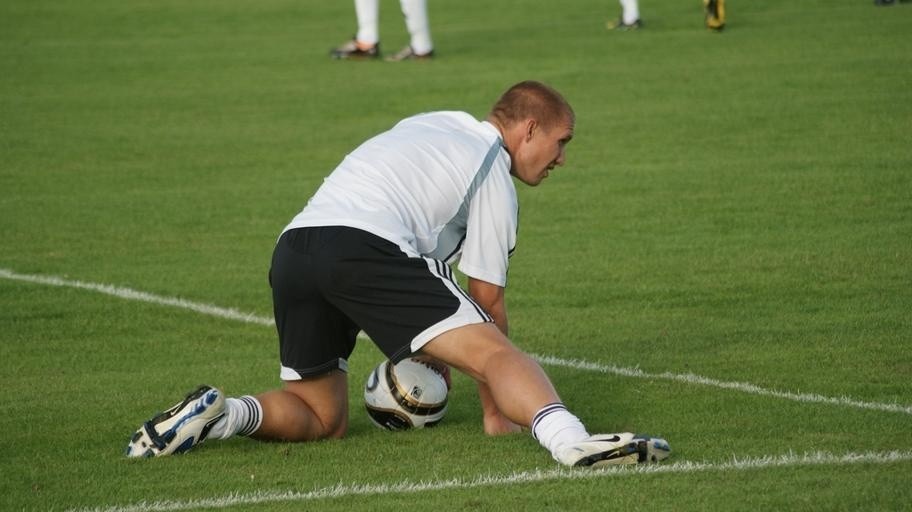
702 0 727 32
127 80 672 471
328 0 437 62
605 0 642 32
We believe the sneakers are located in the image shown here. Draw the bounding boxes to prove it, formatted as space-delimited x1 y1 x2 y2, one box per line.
329 35 380 59
124 384 227 460
605 15 641 31
565 430 671 470
386 45 436 60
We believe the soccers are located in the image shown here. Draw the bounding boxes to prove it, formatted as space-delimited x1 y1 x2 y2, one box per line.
364 352 452 433
